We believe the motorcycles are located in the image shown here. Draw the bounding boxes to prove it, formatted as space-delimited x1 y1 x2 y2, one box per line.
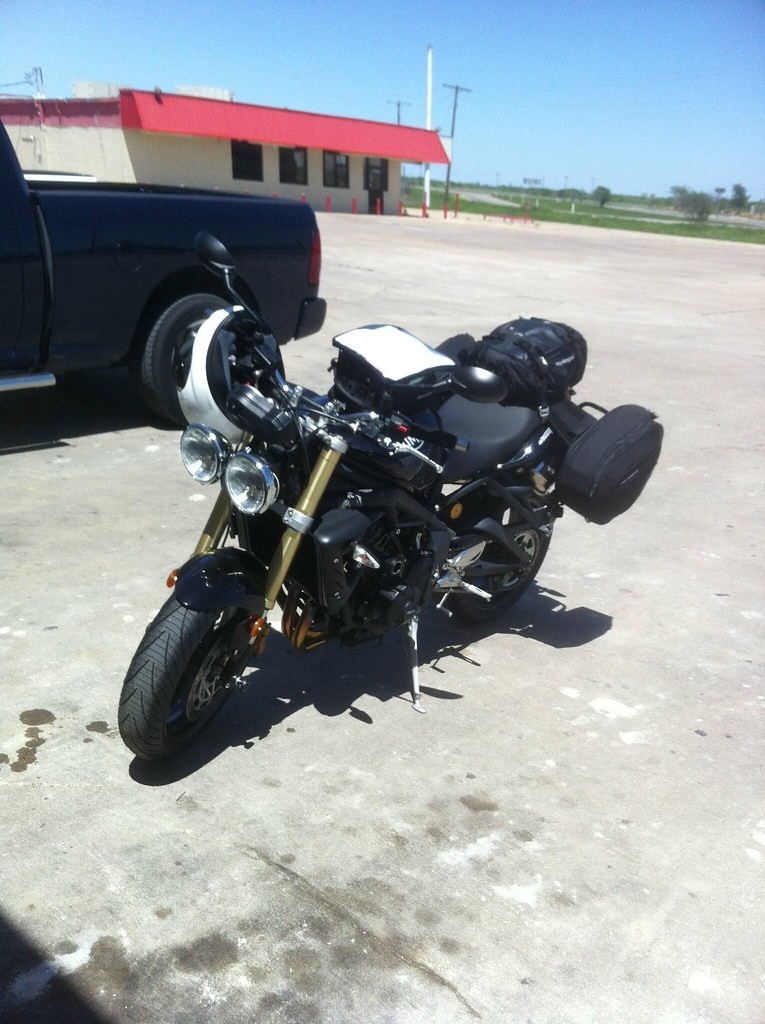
120 229 663 760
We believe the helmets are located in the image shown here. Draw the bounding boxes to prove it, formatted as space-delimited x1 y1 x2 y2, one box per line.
167 305 285 447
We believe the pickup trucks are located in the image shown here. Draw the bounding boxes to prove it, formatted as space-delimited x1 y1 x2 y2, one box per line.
0 117 327 428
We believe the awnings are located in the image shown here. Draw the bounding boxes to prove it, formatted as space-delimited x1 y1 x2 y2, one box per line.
119 88 449 163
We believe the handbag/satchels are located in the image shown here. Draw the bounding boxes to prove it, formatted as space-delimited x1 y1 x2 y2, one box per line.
467 315 588 420
554 404 664 525
